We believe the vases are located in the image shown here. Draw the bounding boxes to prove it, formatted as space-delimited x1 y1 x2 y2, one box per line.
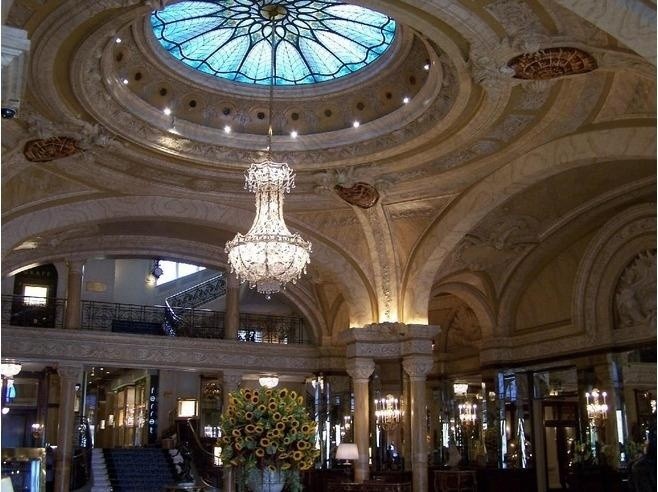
248 467 286 492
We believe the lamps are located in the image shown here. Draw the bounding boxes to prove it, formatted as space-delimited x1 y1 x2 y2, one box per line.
336 444 359 465
1 359 22 377
259 375 279 388
223 10 313 300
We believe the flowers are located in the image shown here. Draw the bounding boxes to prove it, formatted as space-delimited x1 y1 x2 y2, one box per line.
220 385 321 473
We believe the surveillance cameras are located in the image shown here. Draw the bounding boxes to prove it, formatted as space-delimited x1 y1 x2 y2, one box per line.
1 107 16 119
